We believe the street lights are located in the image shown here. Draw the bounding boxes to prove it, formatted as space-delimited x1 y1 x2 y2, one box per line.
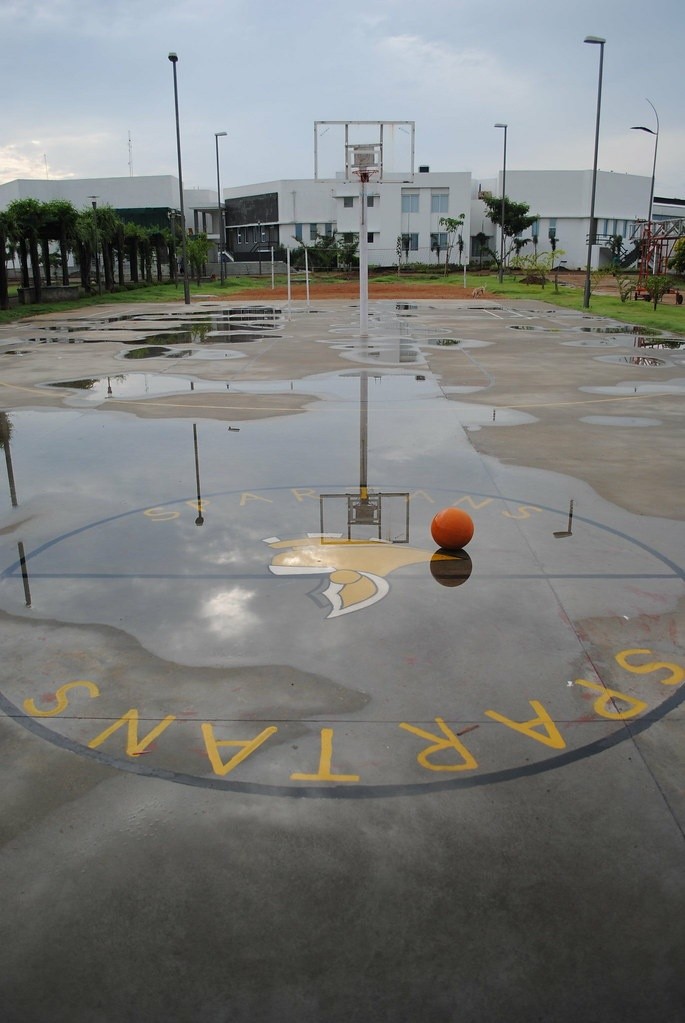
553 500 574 539
495 124 508 285
227 384 240 432
583 35 606 308
169 52 190 303
256 219 263 274
215 132 227 286
168 209 181 289
87 196 102 296
104 377 116 399
191 382 204 526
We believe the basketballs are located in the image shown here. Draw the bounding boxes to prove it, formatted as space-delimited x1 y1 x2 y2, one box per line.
430 506 474 549
430 547 473 588
